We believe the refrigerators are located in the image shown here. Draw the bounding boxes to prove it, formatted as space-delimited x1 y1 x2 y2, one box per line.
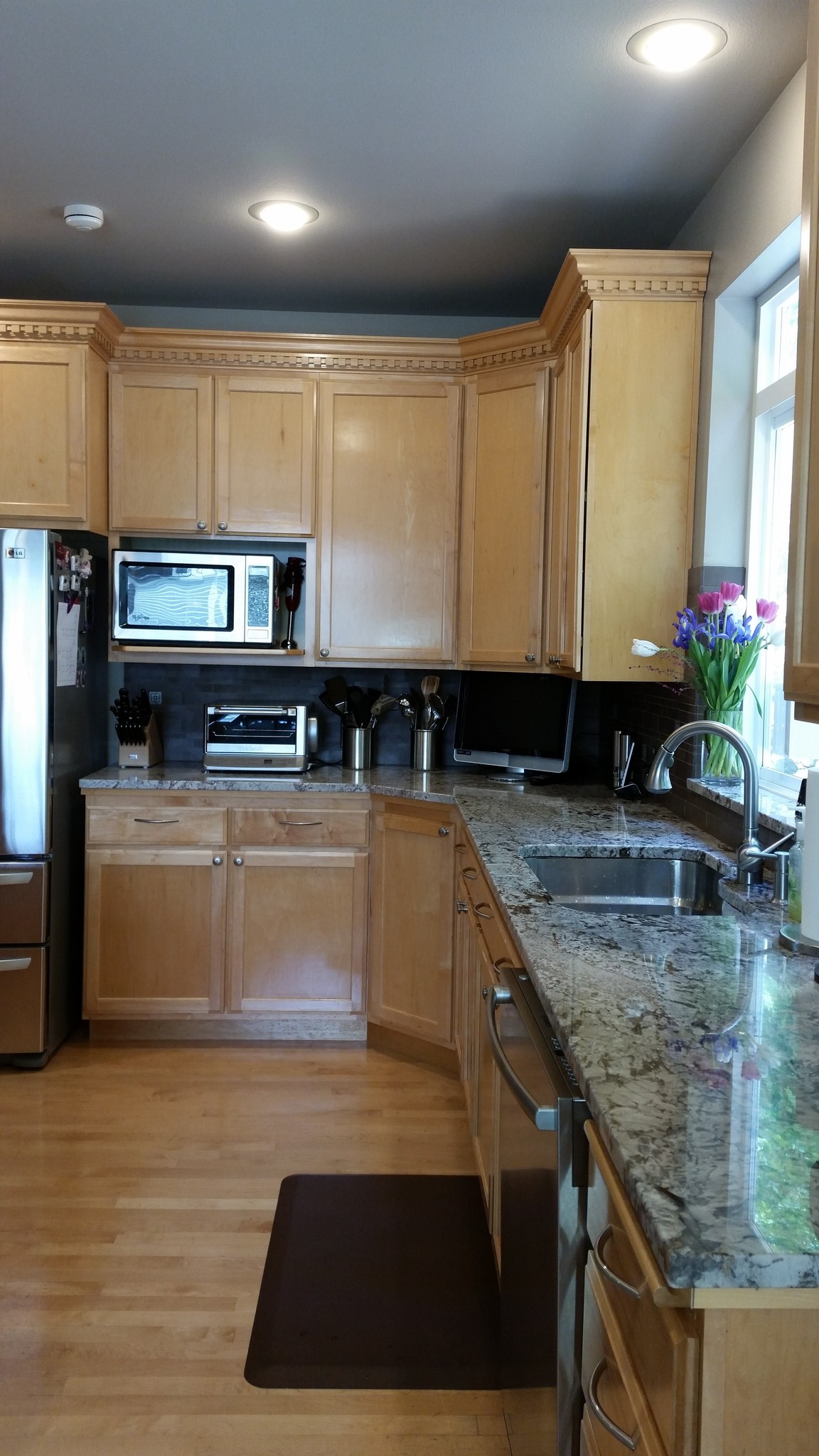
0 526 102 1069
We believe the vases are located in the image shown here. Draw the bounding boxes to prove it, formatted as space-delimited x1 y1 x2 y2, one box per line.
699 709 744 786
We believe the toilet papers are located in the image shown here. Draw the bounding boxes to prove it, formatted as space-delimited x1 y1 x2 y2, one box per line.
800 766 819 942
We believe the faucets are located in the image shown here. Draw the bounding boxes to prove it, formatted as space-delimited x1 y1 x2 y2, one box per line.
645 721 795 884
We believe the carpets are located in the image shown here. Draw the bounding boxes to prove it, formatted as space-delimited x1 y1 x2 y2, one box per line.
243 1172 496 1392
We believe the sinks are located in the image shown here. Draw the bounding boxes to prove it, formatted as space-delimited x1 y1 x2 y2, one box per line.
517 842 752 917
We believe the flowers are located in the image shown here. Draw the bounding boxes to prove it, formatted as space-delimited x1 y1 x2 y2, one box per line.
630 580 784 782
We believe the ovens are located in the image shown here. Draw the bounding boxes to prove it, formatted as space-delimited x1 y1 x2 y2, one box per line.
201 700 319 775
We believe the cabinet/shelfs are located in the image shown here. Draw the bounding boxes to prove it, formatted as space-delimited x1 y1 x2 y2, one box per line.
574 1117 819 1456
0 300 125 538
105 326 318 541
781 0 819 722
81 787 527 1276
543 243 715 685
306 335 458 672
458 317 573 677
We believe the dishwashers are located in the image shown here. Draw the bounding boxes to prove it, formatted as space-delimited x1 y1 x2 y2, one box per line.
481 966 593 1456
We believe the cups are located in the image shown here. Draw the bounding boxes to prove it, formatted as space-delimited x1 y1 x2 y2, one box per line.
341 726 376 770
409 728 446 771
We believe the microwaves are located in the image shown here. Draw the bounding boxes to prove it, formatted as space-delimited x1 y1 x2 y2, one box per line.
109 548 282 649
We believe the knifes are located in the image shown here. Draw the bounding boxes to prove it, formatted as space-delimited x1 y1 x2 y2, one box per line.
109 688 152 747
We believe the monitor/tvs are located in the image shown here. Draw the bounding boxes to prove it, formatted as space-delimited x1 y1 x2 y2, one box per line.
453 671 579 781
